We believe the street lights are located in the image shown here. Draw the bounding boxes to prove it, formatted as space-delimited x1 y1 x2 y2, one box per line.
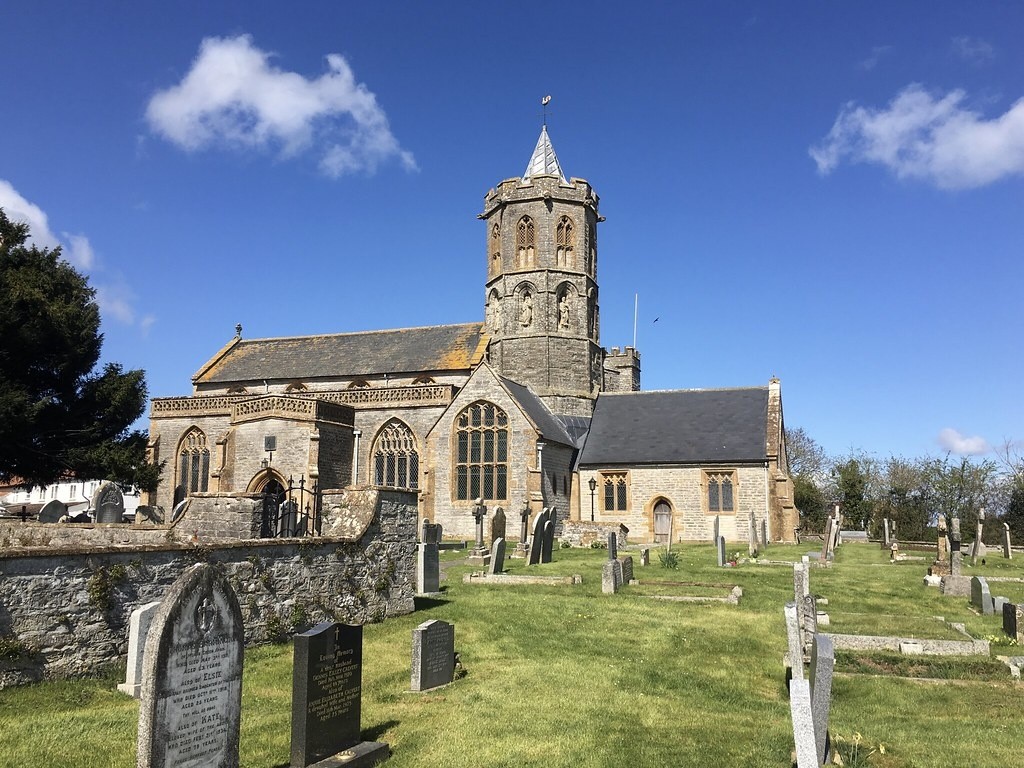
588 475 596 520
